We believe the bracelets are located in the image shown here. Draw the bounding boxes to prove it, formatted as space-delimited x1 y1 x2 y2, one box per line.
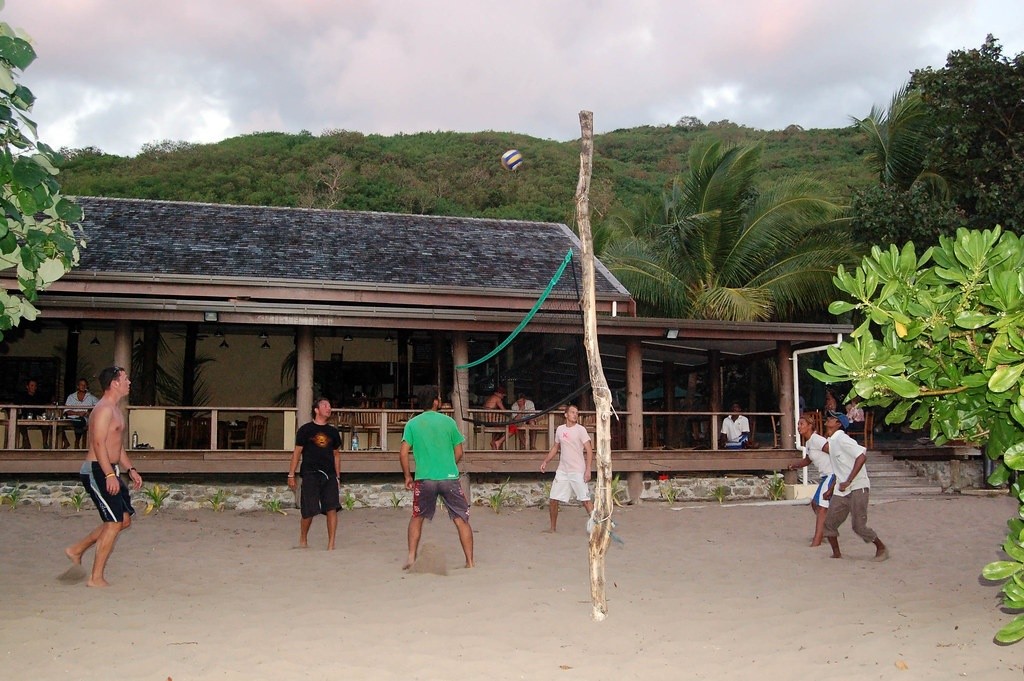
106 473 115 479
127 467 136 472
288 475 294 478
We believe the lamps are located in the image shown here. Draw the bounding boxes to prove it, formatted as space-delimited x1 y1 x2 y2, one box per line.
203 311 219 322
215 324 224 337
467 333 478 342
261 339 270 348
71 322 81 334
344 334 353 341
135 331 144 346
259 329 268 338
219 338 230 348
89 330 101 345
665 328 680 340
384 329 394 342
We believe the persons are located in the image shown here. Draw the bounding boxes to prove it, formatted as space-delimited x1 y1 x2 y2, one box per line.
823 408 889 562
719 400 752 450
787 414 836 547
9 378 51 450
478 386 517 450
287 398 343 552
541 405 594 536
354 390 367 409
399 385 474 569
510 391 538 450
64 366 143 588
799 389 866 446
56 379 100 449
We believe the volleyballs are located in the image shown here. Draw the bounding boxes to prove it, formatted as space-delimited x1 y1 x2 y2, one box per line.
501 149 523 172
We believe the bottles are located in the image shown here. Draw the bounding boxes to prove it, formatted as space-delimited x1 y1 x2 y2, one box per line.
351 433 358 452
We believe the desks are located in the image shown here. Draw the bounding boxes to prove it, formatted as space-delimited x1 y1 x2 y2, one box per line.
0 419 81 449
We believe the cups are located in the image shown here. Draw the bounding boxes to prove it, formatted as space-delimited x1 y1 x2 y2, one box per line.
27 413 33 419
42 413 47 419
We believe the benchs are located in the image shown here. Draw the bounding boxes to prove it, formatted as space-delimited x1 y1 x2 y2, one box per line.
473 412 652 450
326 395 453 450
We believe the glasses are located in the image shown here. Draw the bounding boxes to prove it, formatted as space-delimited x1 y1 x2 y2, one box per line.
108 366 119 386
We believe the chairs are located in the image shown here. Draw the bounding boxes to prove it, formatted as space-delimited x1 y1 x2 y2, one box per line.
166 414 268 449
771 411 875 449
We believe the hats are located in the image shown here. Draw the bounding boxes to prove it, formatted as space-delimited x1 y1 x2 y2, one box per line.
827 410 849 428
